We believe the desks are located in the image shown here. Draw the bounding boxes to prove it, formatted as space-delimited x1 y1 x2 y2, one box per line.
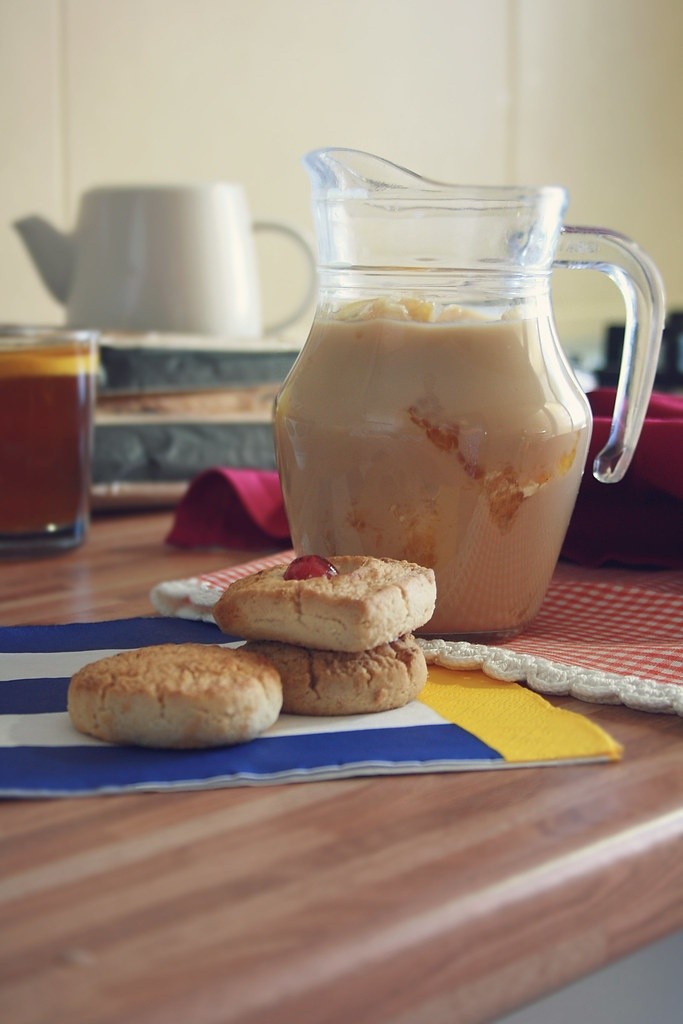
0 496 683 1024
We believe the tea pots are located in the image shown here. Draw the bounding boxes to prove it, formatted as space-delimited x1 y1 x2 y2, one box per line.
14 181 318 342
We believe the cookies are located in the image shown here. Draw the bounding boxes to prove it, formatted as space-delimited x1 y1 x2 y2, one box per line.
211 552 437 652
237 630 428 714
67 642 283 748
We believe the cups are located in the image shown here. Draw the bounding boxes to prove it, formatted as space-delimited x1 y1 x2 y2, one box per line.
0 324 101 552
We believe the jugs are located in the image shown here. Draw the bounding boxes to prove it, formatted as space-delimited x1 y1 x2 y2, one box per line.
274 148 666 643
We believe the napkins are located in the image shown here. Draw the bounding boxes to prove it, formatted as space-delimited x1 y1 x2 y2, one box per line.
160 463 297 549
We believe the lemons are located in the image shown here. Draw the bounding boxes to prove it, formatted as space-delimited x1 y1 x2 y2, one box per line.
0 350 100 377
